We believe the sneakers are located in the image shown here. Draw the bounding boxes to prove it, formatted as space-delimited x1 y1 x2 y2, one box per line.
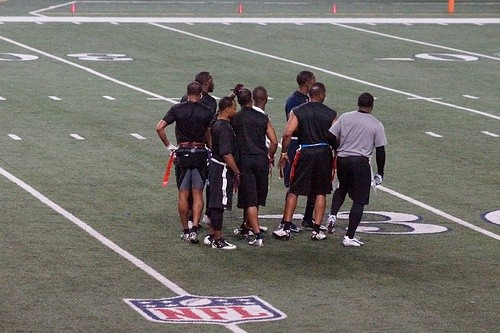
180 232 200 243
204 235 236 250
202 213 212 228
272 227 290 241
234 224 249 239
301 218 327 231
310 230 326 240
187 221 203 231
248 236 265 247
278 221 301 233
249 226 268 235
342 235 364 246
326 215 337 235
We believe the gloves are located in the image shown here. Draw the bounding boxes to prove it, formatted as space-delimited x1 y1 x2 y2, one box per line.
374 173 383 186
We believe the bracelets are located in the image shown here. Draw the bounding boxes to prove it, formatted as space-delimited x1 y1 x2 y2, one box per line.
280 153 287 155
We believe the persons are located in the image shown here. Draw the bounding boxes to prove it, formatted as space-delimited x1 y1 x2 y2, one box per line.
230 89 278 246
155 81 214 243
204 83 244 249
327 92 387 246
272 82 339 240
240 86 271 232
180 71 217 231
285 71 316 232
